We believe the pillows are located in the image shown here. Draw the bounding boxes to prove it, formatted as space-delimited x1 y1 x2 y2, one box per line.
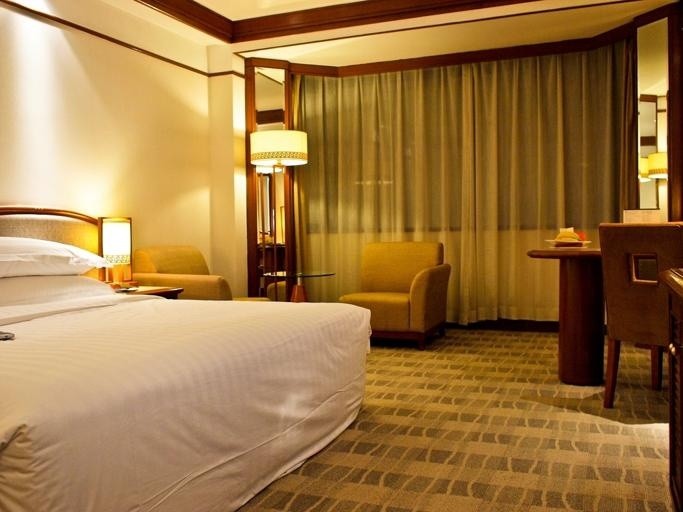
0 234 131 330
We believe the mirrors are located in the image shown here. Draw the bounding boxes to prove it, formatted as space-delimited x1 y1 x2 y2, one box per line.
246 56 297 302
630 13 671 210
638 94 660 210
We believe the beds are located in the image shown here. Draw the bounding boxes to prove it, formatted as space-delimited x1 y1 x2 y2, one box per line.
0 206 373 512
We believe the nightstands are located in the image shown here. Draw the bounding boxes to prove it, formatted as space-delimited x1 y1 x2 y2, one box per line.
120 286 184 300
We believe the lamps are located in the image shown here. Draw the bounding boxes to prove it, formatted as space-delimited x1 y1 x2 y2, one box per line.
249 129 309 303
101 216 134 293
649 149 668 206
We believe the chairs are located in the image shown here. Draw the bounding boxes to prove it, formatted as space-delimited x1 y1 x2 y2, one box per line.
598 210 682 409
130 243 271 302
339 238 453 351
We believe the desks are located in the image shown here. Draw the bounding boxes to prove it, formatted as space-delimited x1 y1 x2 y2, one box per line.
525 242 607 386
